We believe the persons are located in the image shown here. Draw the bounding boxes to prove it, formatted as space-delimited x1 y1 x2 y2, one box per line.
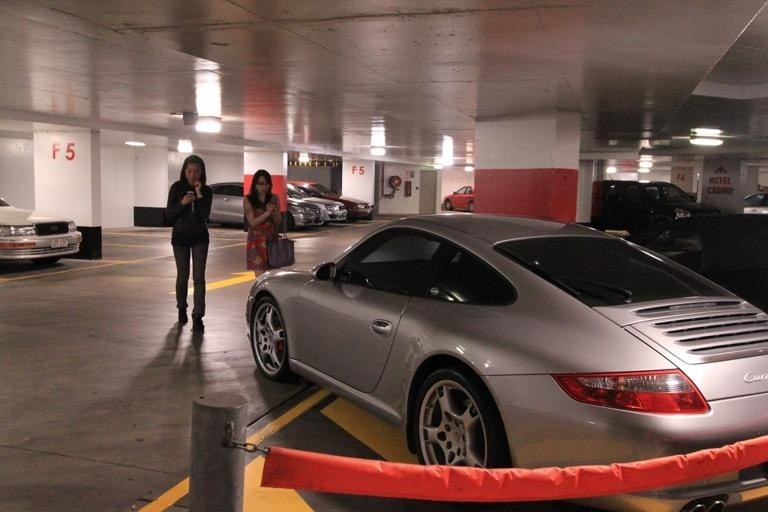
242 169 282 279
163 154 214 332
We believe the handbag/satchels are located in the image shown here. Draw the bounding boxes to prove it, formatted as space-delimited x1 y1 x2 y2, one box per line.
267 221 295 269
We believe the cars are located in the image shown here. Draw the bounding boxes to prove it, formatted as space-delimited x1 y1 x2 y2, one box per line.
441 185 473 212
0 196 84 267
590 174 767 311
204 180 373 230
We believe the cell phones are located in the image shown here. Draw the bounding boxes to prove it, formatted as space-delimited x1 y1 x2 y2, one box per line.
188 192 193 197
272 197 277 204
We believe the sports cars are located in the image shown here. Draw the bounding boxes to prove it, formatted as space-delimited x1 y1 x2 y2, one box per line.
247 212 768 508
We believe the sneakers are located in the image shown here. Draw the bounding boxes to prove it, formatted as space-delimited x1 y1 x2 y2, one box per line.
178 309 188 323
193 318 204 331
